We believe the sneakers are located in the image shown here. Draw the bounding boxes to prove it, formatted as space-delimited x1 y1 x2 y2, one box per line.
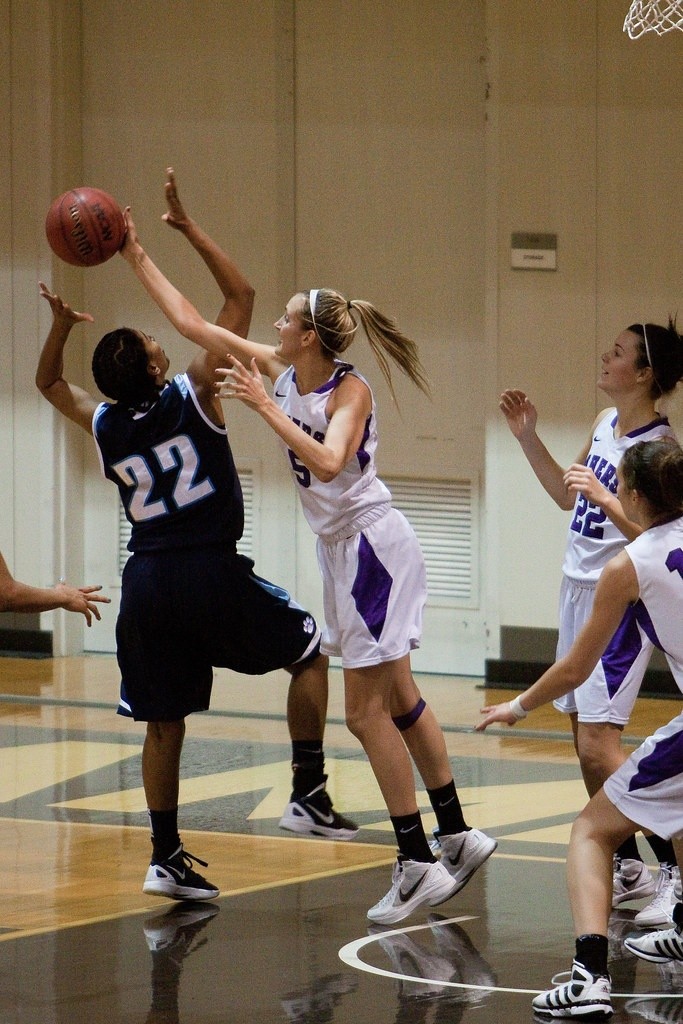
277 971 361 1022
366 913 500 1009
623 928 683 965
146 901 222 955
424 828 500 909
141 843 221 900
622 997 683 1024
530 957 617 1019
277 781 362 843
367 850 459 926
611 854 683 927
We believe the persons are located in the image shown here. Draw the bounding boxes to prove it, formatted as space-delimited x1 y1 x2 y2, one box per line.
475 439 683 1021
500 322 683 927
0 554 111 627
118 205 497 924
35 167 358 901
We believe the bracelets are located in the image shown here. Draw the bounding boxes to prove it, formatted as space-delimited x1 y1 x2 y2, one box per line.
510 696 528 720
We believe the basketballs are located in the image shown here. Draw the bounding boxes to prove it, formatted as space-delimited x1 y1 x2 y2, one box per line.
44 186 126 268
